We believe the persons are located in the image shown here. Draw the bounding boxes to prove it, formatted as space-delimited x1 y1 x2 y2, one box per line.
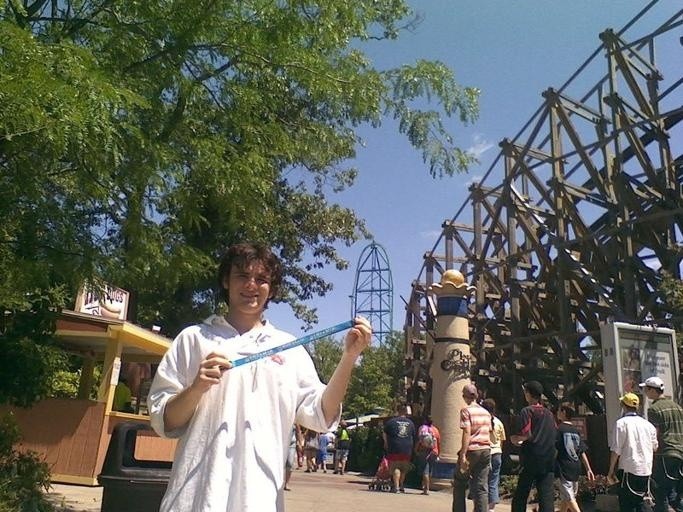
286 418 351 491
607 378 681 510
452 385 507 512
113 375 132 413
382 406 441 496
510 380 596 511
146 241 372 511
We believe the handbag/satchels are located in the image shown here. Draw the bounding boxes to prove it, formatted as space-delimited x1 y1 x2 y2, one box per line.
416 434 434 459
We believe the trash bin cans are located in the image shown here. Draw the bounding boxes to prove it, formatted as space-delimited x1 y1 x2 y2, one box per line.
96 420 173 512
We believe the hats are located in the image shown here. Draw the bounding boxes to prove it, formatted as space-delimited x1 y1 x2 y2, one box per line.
639 377 665 390
619 393 640 407
463 384 477 398
